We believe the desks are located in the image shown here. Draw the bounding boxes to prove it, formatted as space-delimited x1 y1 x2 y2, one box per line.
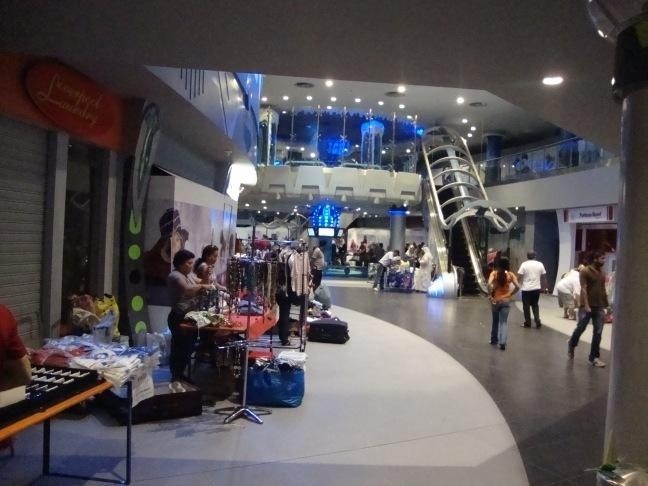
0 380 132 486
179 296 280 382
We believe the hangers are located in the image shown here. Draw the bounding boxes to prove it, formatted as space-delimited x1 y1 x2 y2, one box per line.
232 241 306 264
213 240 272 424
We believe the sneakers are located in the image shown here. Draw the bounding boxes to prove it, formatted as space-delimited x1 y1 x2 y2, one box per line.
568 343 575 359
588 357 607 367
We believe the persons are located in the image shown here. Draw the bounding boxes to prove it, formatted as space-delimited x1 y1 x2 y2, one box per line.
556 264 585 320
567 250 609 368
145 207 189 288
310 240 328 292
165 249 215 384
310 273 332 311
194 258 215 296
201 244 228 293
487 257 519 351
517 250 547 328
338 234 432 292
0 303 32 393
